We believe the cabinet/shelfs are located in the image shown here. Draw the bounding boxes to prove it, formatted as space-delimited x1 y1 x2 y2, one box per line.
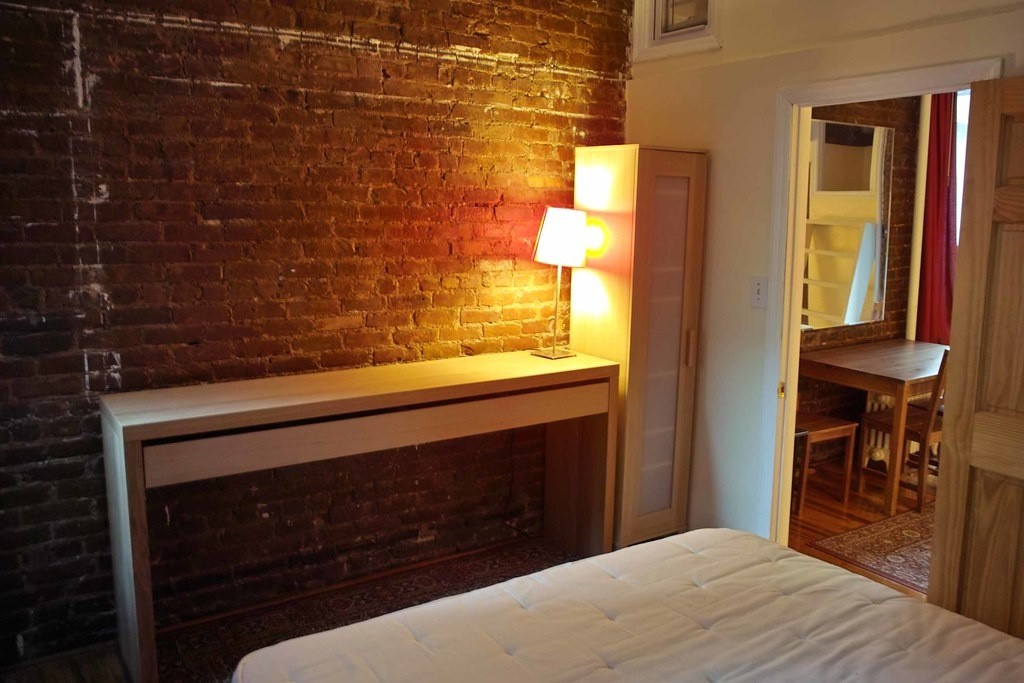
570 142 713 550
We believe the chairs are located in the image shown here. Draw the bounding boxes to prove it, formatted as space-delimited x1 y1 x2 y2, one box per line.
796 413 860 516
856 348 950 514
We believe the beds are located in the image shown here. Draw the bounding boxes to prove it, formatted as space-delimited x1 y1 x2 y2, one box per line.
228 526 1024 683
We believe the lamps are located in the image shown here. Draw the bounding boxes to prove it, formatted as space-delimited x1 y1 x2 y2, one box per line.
529 205 590 360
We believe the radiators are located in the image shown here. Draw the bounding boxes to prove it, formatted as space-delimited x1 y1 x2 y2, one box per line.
861 389 943 473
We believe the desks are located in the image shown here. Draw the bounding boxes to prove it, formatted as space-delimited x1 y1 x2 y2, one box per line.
798 338 951 515
98 346 621 683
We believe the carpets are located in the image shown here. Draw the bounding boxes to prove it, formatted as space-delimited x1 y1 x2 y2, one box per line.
805 497 935 594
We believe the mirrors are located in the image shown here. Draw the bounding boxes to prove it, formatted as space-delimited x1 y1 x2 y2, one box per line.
799 119 896 333
